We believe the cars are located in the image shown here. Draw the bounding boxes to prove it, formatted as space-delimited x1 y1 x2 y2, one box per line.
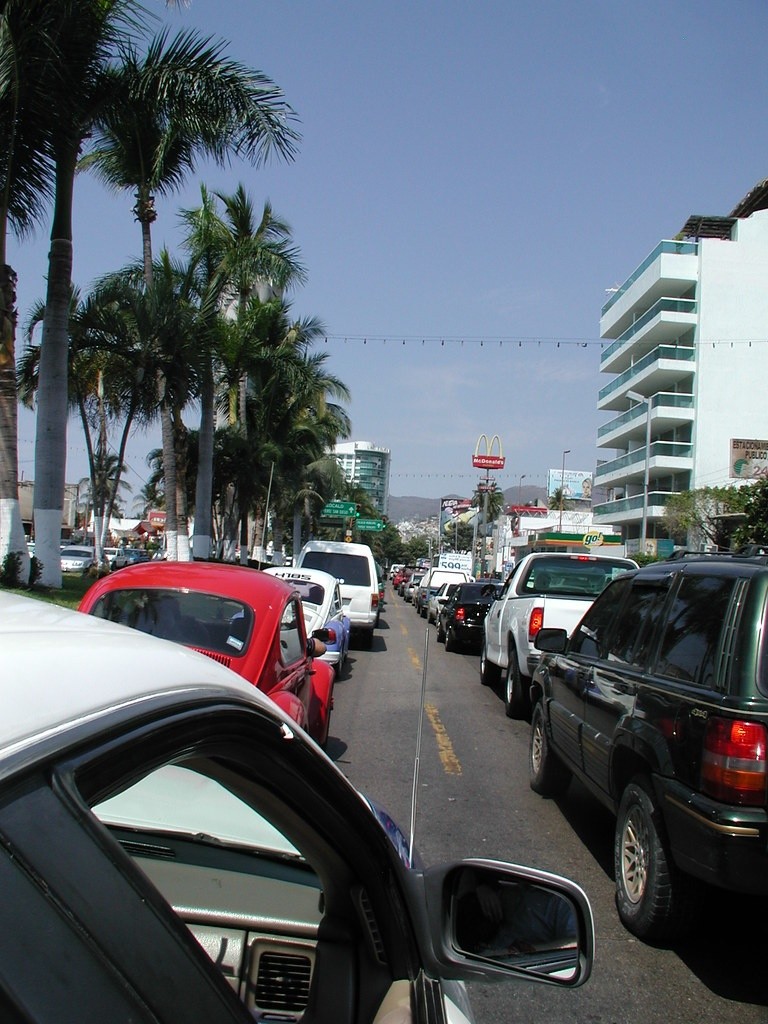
71 558 336 764
388 562 476 625
262 567 352 677
435 581 505 655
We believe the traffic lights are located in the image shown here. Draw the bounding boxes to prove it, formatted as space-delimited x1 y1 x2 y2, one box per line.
355 503 361 518
381 517 387 529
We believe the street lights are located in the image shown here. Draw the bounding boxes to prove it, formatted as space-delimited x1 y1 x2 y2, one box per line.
556 449 572 532
516 475 526 531
624 390 654 558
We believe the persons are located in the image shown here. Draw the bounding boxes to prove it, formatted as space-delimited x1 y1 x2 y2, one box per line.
158 595 204 644
306 638 327 657
581 479 592 498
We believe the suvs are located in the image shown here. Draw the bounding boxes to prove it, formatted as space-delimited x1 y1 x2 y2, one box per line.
527 542 768 951
476 551 642 722
0 584 597 1024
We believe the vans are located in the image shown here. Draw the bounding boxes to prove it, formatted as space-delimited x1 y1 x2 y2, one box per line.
291 540 381 650
